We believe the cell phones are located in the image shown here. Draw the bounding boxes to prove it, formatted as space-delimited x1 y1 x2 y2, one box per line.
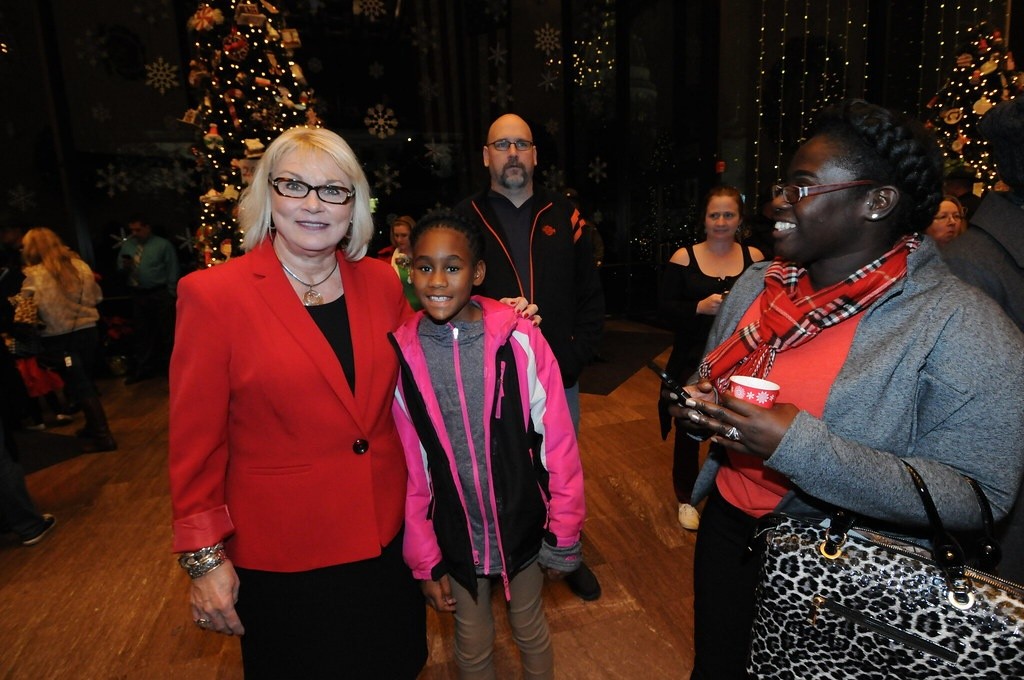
646 358 705 417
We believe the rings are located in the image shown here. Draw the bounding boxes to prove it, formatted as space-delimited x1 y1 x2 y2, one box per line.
725 427 740 441
197 618 207 627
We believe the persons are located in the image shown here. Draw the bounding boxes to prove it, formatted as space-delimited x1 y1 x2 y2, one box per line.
377 216 423 311
0 215 116 544
167 124 544 680
453 113 606 602
387 209 587 680
117 212 180 384
653 99 1024 680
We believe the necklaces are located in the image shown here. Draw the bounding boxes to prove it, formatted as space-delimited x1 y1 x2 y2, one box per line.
280 262 338 307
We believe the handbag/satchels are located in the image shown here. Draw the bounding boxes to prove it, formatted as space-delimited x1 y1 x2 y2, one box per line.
735 457 1024 680
60 350 83 378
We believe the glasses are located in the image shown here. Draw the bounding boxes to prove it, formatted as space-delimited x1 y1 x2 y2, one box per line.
264 171 360 205
762 178 879 205
933 212 965 224
483 138 536 152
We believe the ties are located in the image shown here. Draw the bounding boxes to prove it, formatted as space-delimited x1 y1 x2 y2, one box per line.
130 242 146 288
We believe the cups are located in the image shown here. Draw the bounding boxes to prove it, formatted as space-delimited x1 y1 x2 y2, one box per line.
729 375 780 410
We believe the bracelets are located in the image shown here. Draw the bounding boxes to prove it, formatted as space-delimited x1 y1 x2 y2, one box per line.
180 543 226 578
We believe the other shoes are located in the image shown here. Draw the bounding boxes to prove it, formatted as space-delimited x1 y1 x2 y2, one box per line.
21 512 56 548
564 560 604 602
677 502 700 531
12 410 48 431
66 423 115 454
